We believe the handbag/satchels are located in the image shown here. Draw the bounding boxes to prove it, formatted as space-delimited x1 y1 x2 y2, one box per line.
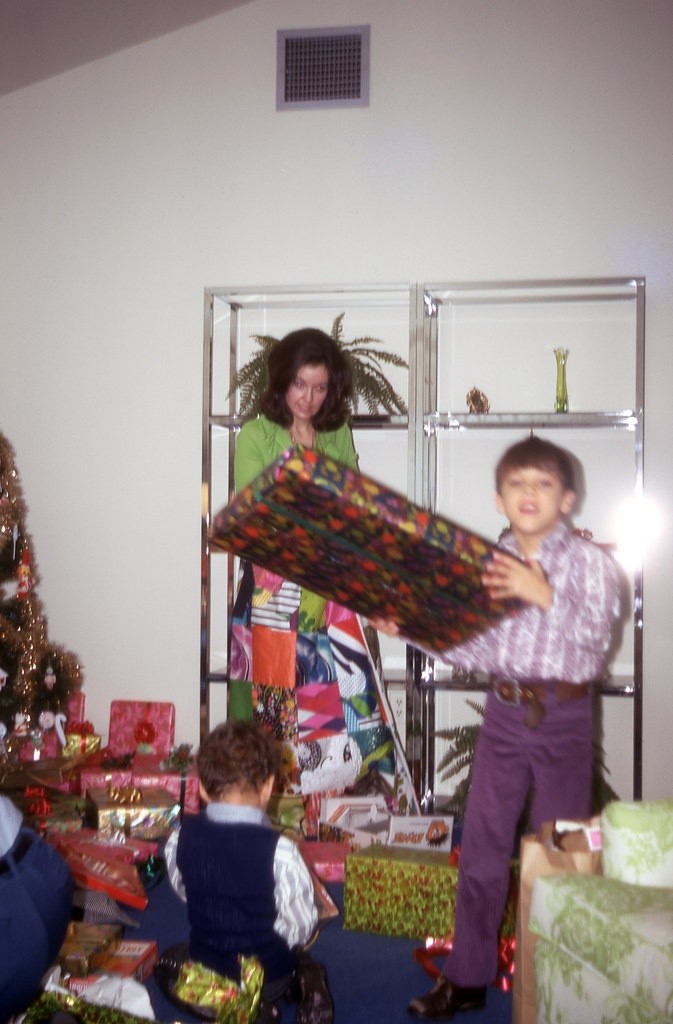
288 944 334 1024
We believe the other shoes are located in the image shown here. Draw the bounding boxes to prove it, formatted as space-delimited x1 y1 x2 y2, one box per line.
408 974 487 1020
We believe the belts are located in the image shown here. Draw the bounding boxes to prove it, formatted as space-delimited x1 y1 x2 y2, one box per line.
1 817 41 872
487 671 595 706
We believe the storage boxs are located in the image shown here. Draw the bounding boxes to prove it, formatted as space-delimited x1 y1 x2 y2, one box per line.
19 692 671 1021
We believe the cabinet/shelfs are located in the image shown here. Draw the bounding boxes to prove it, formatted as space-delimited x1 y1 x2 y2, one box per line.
195 273 651 690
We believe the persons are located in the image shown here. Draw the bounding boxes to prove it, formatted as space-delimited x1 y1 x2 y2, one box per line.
161 717 318 1006
234 325 417 836
0 792 73 1021
364 437 624 1021
